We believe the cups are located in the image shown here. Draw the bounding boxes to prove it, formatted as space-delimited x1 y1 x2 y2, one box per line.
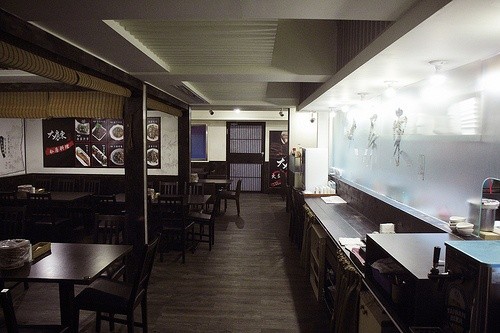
315 186 335 194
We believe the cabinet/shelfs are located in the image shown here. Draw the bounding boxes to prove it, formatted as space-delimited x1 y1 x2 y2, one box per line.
208 173 228 191
357 292 398 333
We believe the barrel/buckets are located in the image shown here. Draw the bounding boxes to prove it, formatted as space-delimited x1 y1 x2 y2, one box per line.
469 198 499 231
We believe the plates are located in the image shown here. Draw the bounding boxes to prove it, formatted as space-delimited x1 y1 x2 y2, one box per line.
146 148 159 166
76 147 90 166
110 149 124 166
109 124 124 141
92 125 106 140
75 120 90 135
92 145 107 166
147 124 158 142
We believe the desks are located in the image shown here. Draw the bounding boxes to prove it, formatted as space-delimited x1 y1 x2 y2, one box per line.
304 196 413 333
0 243 134 333
100 193 211 240
198 179 234 211
0 191 94 233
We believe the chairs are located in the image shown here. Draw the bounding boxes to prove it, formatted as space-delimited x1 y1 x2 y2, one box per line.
0 191 73 290
184 182 205 195
188 197 218 250
280 170 305 252
73 232 161 333
218 180 241 216
89 194 152 245
157 196 194 264
34 178 101 225
0 288 71 333
159 181 178 195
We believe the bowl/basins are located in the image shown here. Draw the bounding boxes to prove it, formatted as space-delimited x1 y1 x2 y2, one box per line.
449 216 474 236
